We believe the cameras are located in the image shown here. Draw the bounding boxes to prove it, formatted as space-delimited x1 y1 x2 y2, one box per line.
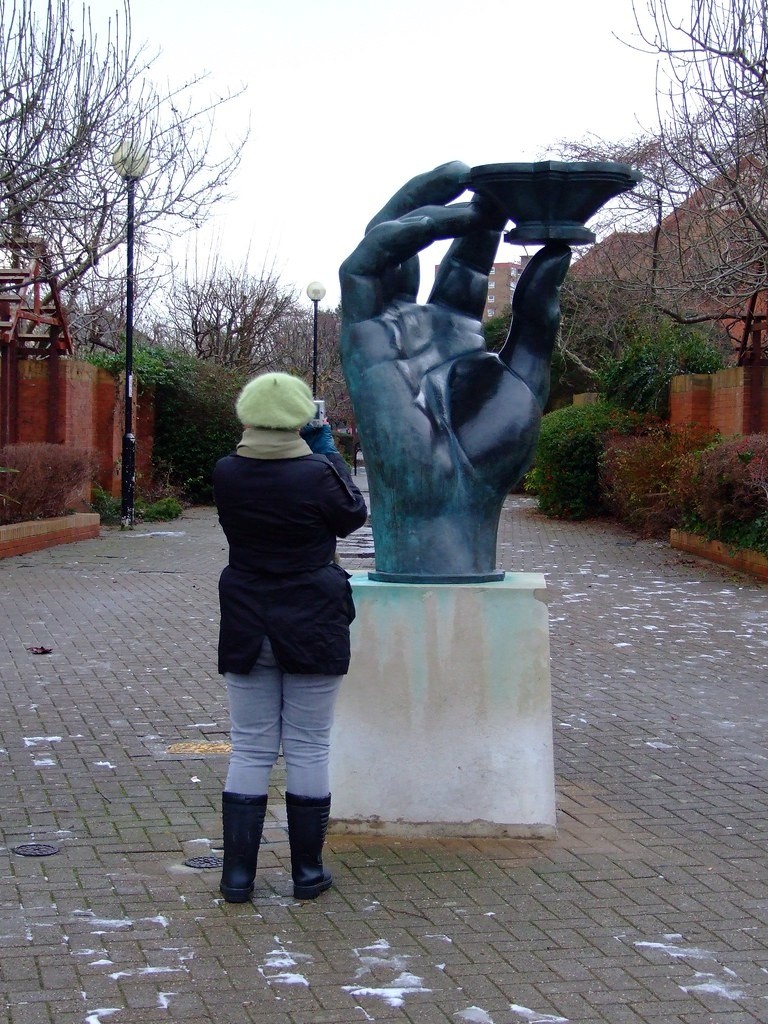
302 400 325 428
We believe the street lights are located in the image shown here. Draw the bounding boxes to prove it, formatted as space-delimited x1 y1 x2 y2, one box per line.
305 282 326 400
110 142 150 529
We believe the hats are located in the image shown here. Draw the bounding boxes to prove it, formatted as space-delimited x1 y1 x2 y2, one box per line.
235 371 316 429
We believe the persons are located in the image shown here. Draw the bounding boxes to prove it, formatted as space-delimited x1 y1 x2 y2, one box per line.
335 158 574 574
212 372 370 906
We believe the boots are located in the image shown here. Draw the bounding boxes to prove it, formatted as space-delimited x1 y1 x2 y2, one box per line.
220 791 268 903
285 791 332 900
